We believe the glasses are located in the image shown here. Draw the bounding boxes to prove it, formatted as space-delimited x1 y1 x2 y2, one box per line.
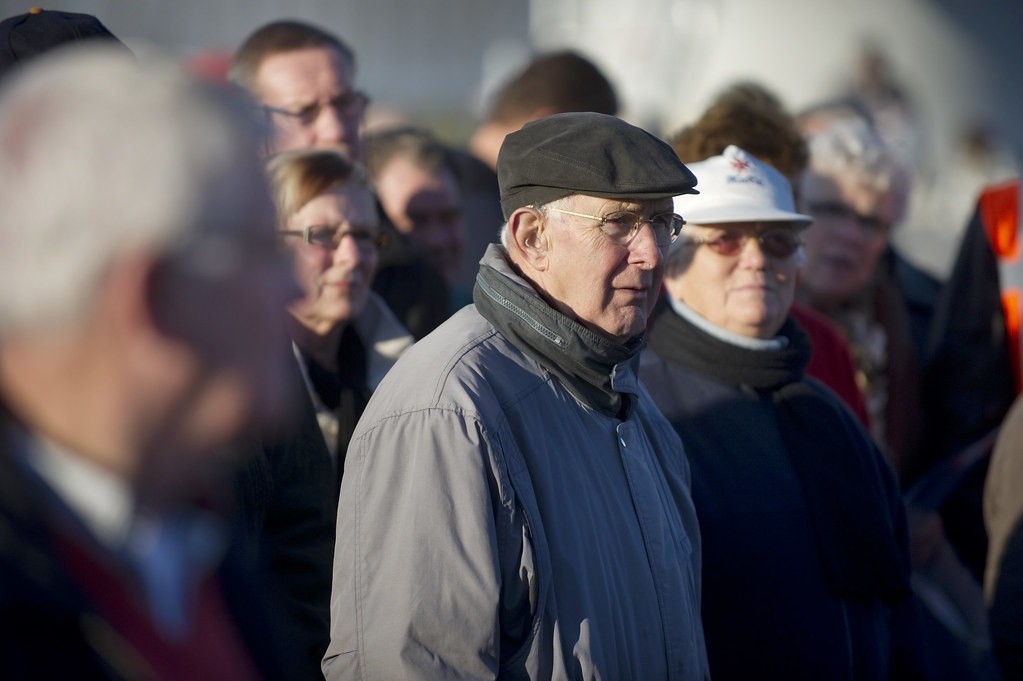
276 222 393 251
667 227 805 259
801 196 888 233
261 90 369 125
526 204 687 247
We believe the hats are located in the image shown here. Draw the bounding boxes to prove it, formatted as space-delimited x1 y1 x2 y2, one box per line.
670 144 815 225
496 112 701 224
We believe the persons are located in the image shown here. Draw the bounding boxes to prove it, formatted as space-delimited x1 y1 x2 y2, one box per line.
322 113 712 681
0 7 1023 681
